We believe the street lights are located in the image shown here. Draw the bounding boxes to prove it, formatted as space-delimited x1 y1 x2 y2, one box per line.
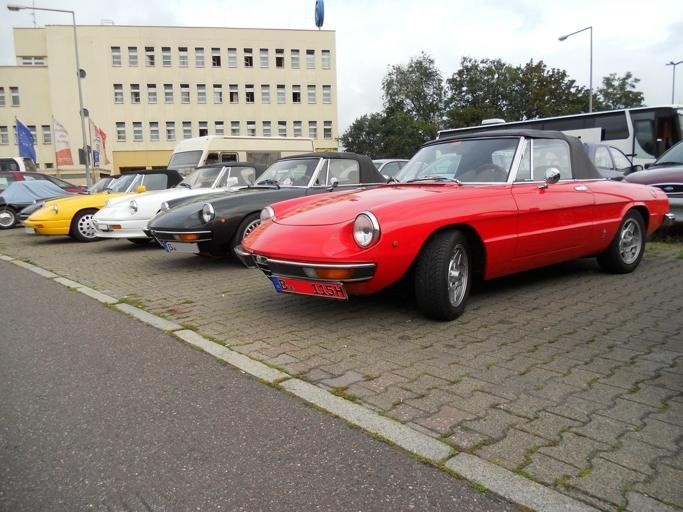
7 6 91 186
558 26 592 111
666 61 683 104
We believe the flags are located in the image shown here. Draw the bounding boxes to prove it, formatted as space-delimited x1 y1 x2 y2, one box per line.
54 121 74 166
89 119 113 170
15 117 36 164
